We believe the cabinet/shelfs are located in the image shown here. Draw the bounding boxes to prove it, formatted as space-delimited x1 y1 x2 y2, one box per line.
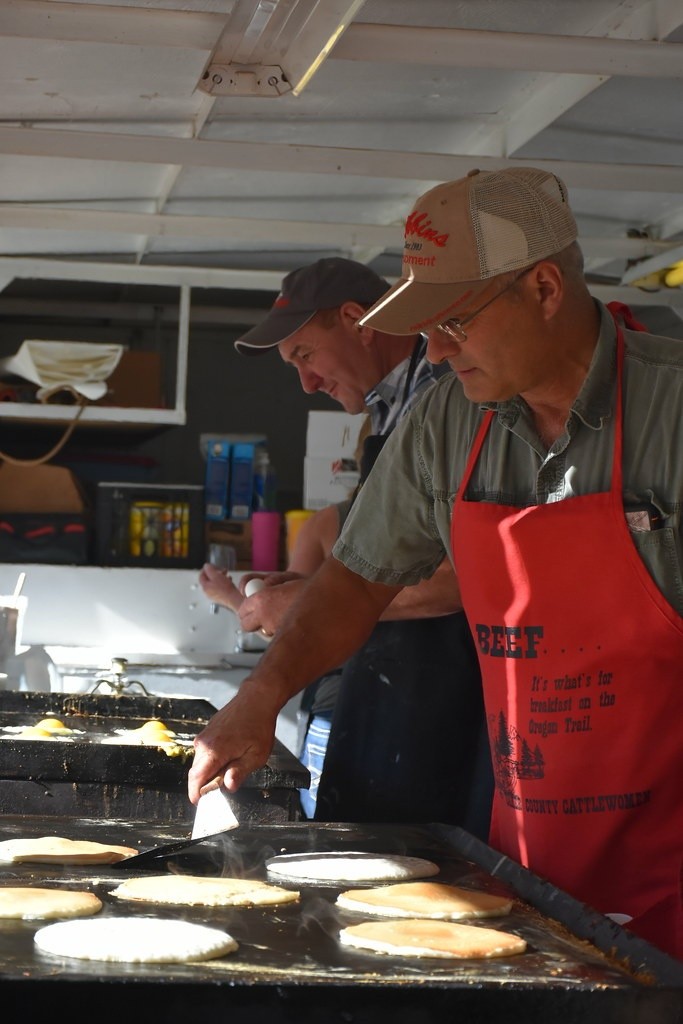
0 256 368 572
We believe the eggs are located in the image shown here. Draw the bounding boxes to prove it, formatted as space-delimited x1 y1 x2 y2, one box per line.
101 721 195 747
1 719 82 741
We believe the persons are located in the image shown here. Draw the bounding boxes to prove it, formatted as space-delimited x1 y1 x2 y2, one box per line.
199 258 495 842
188 166 683 958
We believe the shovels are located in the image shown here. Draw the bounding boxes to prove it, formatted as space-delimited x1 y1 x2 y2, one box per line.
189 771 239 841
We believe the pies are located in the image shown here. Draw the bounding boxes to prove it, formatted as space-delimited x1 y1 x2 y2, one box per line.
335 883 512 922
338 920 528 958
109 876 299 908
265 851 438 883
0 835 138 865
1 887 102 922
35 918 236 966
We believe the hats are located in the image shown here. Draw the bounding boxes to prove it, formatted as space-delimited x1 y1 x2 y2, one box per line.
234 257 392 357
354 167 578 336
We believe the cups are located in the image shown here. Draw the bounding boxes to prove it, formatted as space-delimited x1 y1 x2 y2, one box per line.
286 509 317 565
251 511 281 571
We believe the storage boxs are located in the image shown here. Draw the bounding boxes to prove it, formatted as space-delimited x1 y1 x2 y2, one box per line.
0 339 126 402
95 481 204 570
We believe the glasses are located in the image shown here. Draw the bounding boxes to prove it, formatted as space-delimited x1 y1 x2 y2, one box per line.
419 265 535 343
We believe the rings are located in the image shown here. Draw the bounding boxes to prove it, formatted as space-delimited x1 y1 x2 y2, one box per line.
262 628 273 637
